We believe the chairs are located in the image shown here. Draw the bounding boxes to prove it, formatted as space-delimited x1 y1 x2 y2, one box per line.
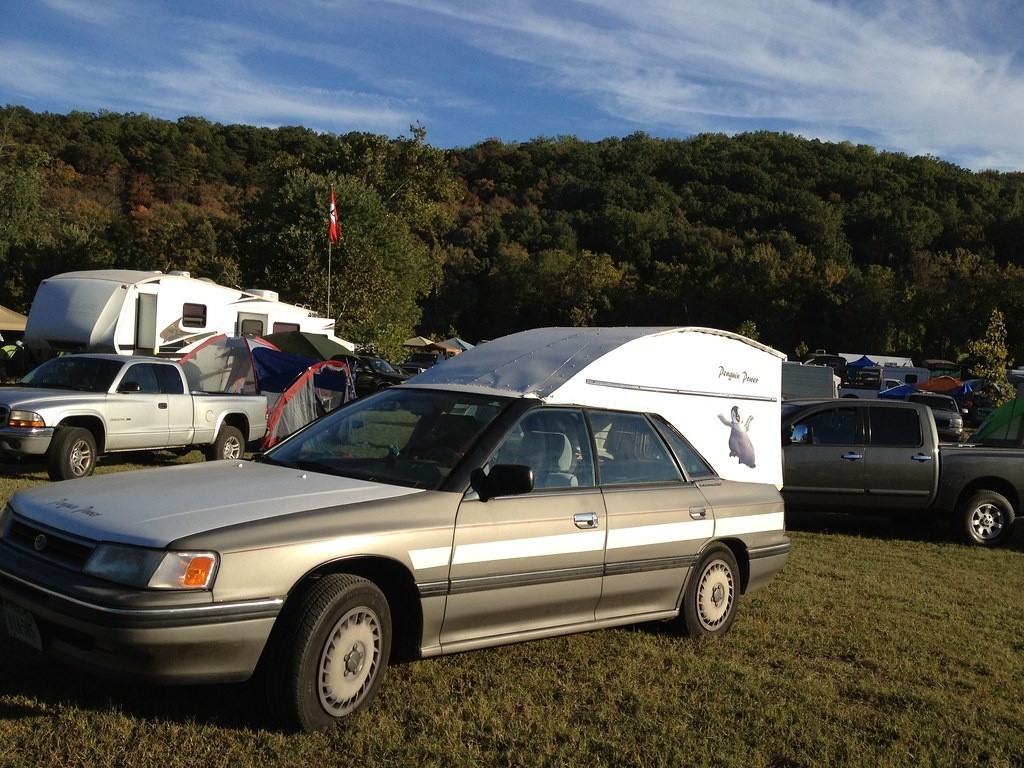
406 415 478 462
519 431 579 487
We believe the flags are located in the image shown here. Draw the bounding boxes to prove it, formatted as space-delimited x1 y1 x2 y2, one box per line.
329 186 340 246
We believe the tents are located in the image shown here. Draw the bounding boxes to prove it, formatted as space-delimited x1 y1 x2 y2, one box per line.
0 305 28 331
169 331 475 452
847 354 878 380
968 394 1024 447
878 374 974 402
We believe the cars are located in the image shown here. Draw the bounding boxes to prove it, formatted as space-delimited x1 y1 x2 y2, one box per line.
0 377 795 743
903 392 969 443
329 352 445 413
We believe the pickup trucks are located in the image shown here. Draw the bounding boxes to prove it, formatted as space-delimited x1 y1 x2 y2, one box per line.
782 396 1024 550
0 352 271 484
